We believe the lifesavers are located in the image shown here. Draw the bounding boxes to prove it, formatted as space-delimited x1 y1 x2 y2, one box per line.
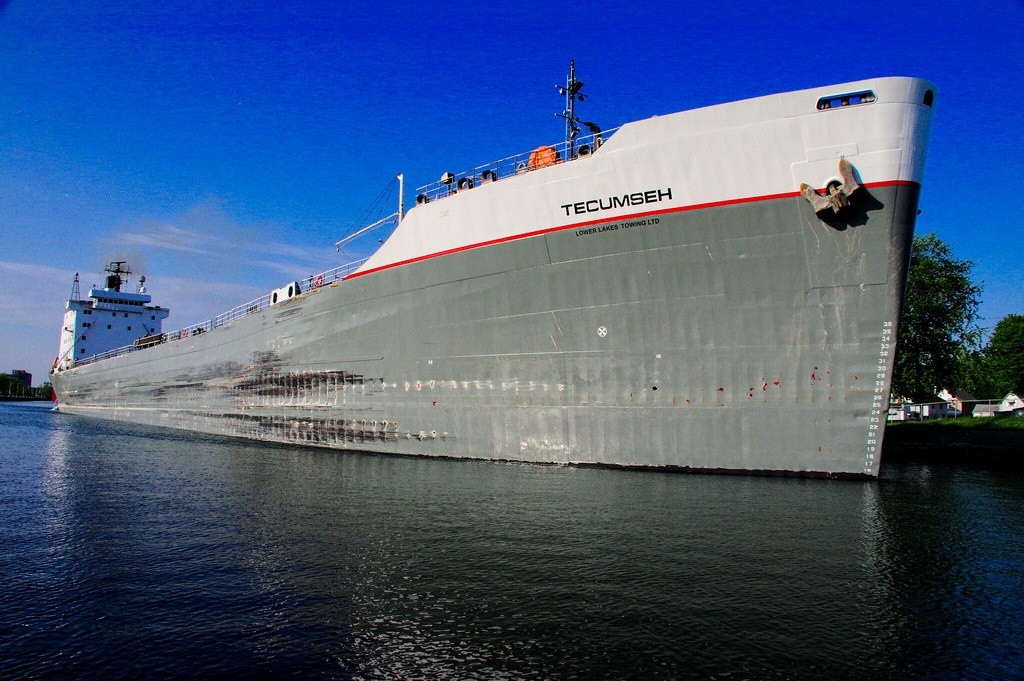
315 275 324 287
183 327 187 336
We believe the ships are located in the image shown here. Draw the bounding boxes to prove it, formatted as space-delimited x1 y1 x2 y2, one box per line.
49 60 935 480
